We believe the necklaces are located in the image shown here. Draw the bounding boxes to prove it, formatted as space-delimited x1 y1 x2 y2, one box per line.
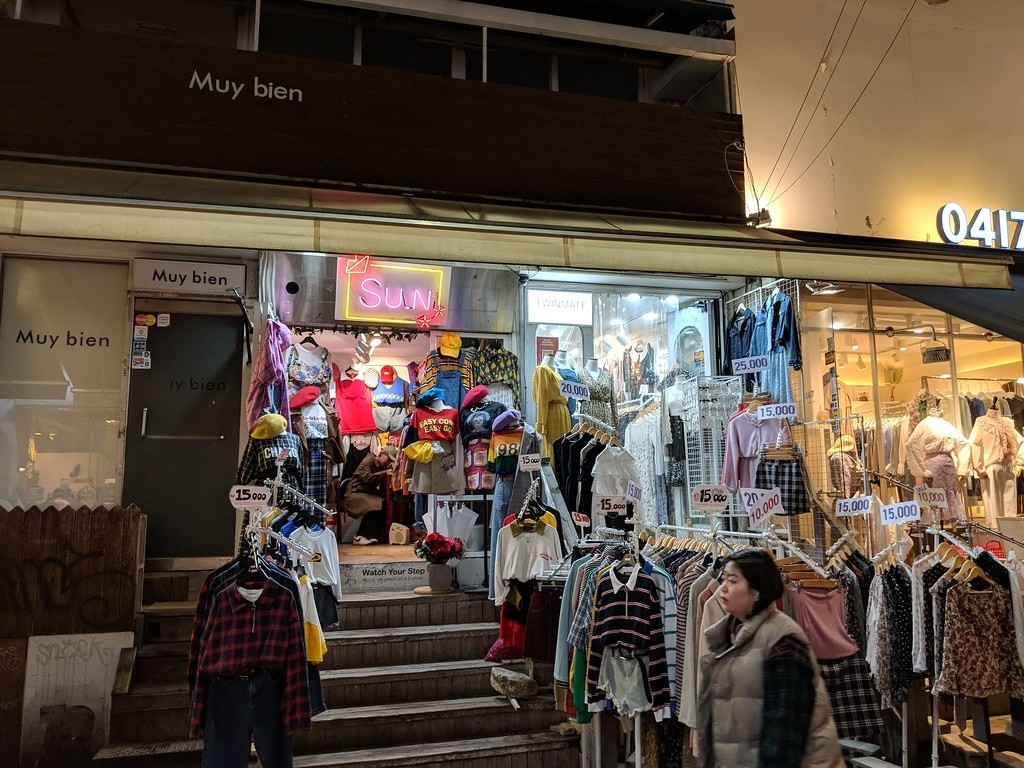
470 400 492 412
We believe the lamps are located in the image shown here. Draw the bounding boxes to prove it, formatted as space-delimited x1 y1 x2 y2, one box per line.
617 335 629 346
806 280 846 295
750 210 772 226
838 334 866 372
897 338 907 349
347 333 418 376
884 324 950 364
599 340 612 353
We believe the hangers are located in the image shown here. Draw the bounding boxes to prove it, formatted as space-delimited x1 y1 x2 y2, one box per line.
616 396 658 423
639 523 776 581
824 533 855 573
345 366 353 372
277 483 328 530
761 428 802 462
933 529 995 593
535 560 571 591
589 526 648 570
238 524 271 584
743 385 771 414
852 405 907 424
870 471 896 501
930 378 1014 398
636 337 645 347
774 542 841 593
270 307 278 321
263 526 307 577
737 296 747 314
771 281 779 299
566 412 634 462
489 339 502 350
1006 537 1018 562
301 329 318 347
516 484 546 523
873 543 903 575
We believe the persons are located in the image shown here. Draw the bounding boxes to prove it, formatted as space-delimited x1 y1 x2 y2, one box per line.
234 413 305 560
341 445 397 545
829 405 1024 548
408 349 622 600
693 548 846 768
289 386 348 527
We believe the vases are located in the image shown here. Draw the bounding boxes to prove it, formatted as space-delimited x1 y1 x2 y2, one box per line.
427 563 453 590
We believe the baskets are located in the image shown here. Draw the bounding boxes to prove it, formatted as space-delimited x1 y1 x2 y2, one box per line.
946 536 1004 559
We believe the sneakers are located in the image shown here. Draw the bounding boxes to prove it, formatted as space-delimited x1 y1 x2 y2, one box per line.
352 534 371 545
368 538 378 544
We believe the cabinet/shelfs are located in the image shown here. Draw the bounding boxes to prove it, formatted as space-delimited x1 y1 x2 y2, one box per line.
680 374 743 517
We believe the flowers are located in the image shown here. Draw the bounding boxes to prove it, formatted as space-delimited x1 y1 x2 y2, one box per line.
414 533 466 565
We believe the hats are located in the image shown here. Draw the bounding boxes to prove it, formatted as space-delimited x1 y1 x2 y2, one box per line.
439 332 462 358
380 446 398 461
491 408 522 432
364 367 379 389
381 365 398 383
408 362 419 383
463 384 488 406
827 434 855 459
249 414 287 440
289 386 320 409
416 387 444 408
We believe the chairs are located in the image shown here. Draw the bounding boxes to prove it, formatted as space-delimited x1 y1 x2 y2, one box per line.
336 479 363 543
